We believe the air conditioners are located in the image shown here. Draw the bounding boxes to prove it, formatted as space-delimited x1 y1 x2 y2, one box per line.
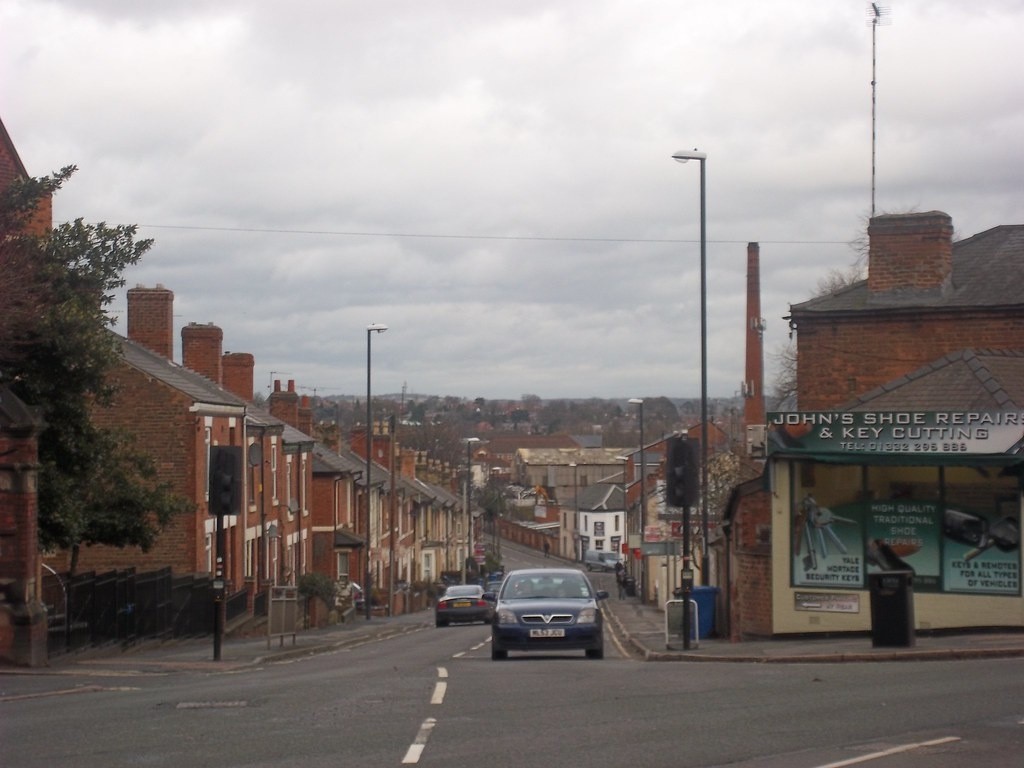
746 424 765 459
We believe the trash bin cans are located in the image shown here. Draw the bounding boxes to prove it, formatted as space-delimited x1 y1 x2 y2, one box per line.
673 585 721 641
624 576 637 597
867 570 916 649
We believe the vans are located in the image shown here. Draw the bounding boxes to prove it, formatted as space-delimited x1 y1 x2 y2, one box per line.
584 550 623 574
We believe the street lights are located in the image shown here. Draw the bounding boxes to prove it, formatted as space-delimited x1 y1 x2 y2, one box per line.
629 398 646 605
671 148 711 587
492 467 501 562
365 322 389 621
570 462 579 561
467 438 481 558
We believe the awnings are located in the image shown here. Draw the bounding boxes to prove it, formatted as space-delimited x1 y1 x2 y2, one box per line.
336 532 367 547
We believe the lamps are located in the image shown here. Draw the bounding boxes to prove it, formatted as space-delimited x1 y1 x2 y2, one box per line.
265 522 280 542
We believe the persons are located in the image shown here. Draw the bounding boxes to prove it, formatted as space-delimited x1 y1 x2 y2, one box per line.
616 562 623 577
544 541 550 559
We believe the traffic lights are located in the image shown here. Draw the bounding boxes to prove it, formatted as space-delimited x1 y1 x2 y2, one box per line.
210 444 241 514
667 437 700 508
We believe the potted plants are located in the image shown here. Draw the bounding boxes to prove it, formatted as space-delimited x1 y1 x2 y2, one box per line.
296 572 340 628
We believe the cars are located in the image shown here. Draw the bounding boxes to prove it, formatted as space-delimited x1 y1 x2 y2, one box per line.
485 581 504 595
481 568 610 660
434 584 496 628
351 581 364 611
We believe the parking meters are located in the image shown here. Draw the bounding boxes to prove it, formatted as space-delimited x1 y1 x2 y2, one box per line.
679 567 694 650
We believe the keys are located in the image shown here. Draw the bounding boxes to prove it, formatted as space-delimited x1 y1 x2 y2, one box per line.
812 507 857 558
962 516 1020 562
